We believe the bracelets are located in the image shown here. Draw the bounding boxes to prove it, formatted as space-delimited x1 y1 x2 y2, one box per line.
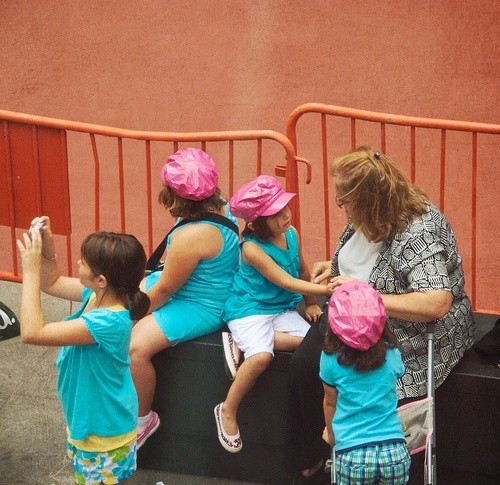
43 254 58 260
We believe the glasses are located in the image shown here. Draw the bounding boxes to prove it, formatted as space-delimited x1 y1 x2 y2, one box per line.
335 194 350 208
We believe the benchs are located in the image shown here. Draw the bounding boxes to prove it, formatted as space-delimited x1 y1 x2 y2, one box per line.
137 295 500 485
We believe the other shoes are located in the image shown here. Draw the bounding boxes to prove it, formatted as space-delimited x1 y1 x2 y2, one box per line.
221 330 240 380
213 401 243 453
136 407 160 450
290 462 325 485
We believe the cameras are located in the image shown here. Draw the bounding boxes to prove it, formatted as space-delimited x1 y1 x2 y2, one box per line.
28 221 46 240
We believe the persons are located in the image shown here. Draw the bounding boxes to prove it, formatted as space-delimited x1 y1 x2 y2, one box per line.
128 147 240 452
16 215 151 485
318 282 412 485
289 145 476 485
213 175 339 454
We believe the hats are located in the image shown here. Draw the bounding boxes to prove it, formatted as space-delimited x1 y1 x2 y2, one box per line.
160 148 219 201
230 175 297 222
328 280 387 351
